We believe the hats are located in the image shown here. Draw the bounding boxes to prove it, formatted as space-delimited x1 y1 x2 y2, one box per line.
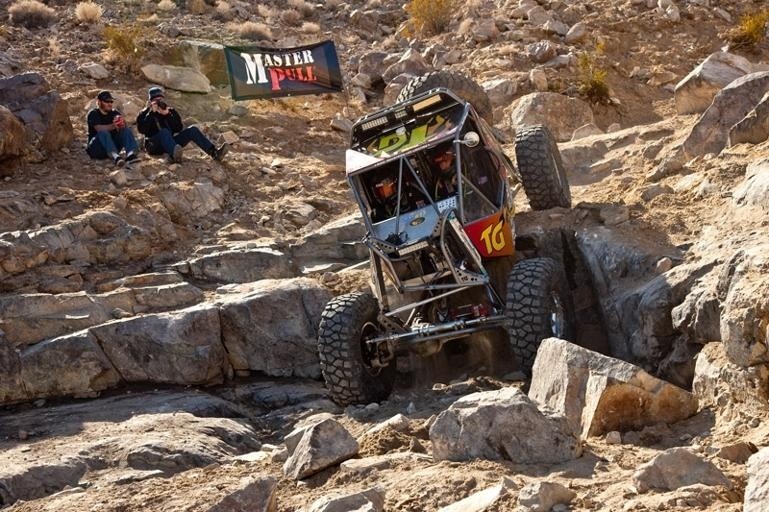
98 91 114 101
149 88 164 100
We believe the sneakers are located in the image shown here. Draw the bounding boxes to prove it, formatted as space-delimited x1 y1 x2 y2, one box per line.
115 156 125 166
173 144 182 164
212 142 228 161
126 153 141 164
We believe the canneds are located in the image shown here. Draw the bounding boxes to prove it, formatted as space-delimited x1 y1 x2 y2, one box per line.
116 115 122 126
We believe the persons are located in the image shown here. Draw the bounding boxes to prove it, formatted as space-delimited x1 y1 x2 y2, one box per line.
375 177 412 219
136 88 230 164
84 89 141 166
436 152 463 197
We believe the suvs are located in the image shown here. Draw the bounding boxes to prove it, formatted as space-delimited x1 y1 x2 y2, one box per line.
317 69 572 407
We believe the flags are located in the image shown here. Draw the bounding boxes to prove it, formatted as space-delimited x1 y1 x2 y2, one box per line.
223 40 342 100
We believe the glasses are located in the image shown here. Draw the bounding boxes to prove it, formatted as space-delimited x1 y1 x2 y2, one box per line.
104 100 113 104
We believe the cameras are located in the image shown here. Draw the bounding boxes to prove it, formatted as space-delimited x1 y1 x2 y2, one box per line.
155 98 167 110
